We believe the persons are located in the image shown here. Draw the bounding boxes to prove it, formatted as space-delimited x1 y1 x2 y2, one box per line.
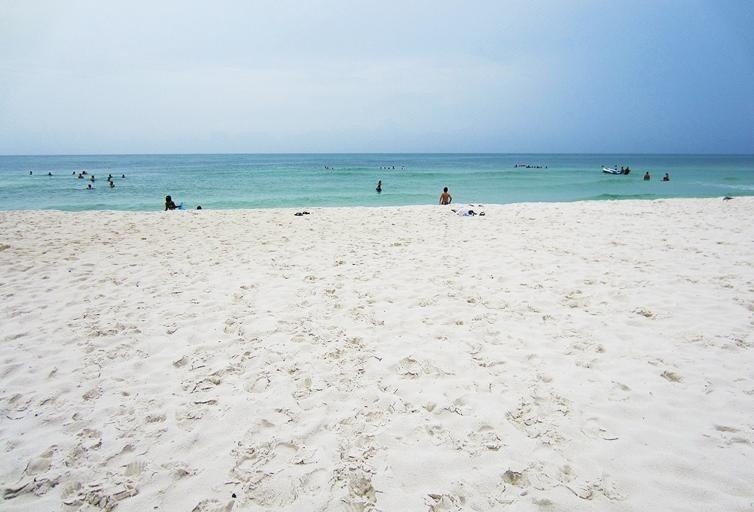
619 167 624 174
376 180 382 192
440 187 452 204
48 172 52 175
644 172 650 180
613 168 618 174
71 170 125 190
663 173 669 180
29 171 32 175
164 196 176 211
624 167 629 174
